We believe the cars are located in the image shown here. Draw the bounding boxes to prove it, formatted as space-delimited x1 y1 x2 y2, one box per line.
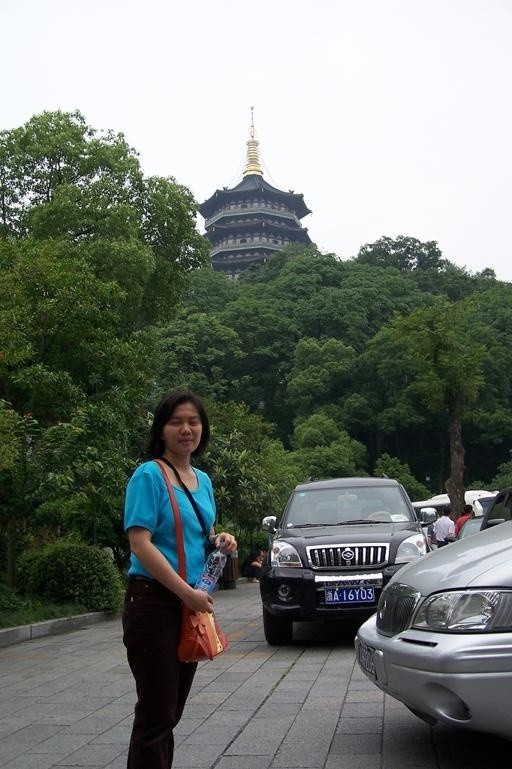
443 515 485 544
463 490 500 518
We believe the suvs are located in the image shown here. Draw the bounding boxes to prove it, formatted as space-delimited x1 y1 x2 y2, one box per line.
354 518 512 743
258 477 439 649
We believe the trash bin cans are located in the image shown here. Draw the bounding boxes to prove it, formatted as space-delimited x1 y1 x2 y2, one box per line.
217 549 239 590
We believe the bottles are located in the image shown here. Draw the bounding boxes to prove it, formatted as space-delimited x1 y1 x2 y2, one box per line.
195 541 227 595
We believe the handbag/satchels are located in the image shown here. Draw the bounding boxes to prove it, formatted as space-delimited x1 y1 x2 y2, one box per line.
177 607 228 664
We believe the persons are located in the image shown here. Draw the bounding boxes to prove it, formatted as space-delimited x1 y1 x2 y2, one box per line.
121 387 239 768
433 505 456 548
241 552 262 583
427 514 440 550
258 550 266 563
455 504 474 540
